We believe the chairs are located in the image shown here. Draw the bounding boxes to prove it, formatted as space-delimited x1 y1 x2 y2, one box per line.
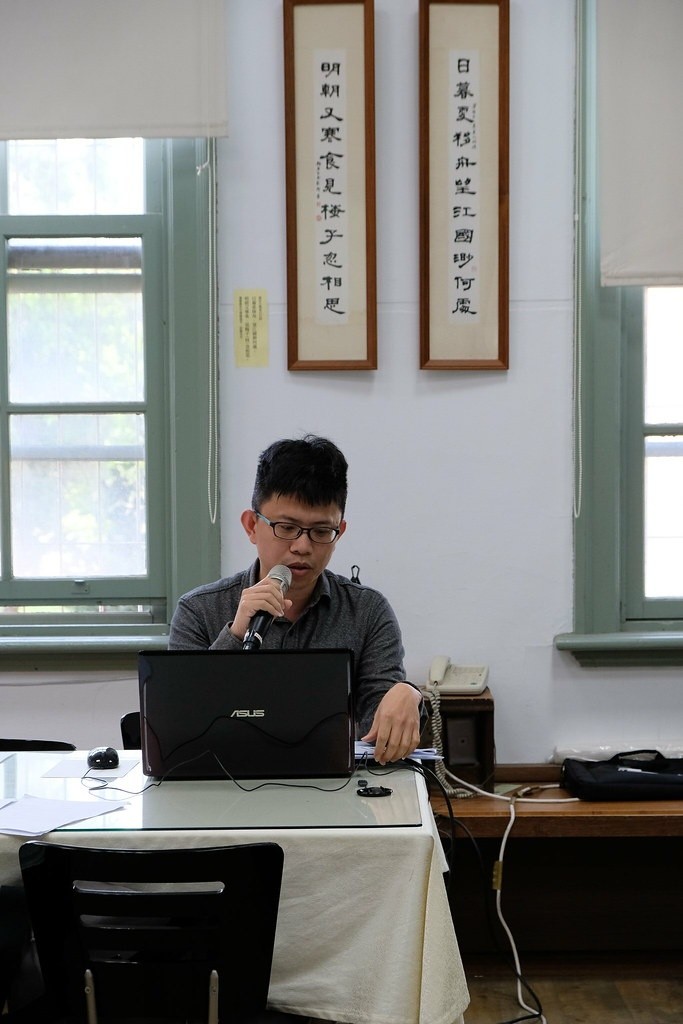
121 711 141 749
19 840 285 1024
0 739 76 751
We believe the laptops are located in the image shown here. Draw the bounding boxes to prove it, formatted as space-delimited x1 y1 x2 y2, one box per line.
138 647 354 781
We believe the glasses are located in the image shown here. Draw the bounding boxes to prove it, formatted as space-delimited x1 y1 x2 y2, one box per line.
253 508 341 544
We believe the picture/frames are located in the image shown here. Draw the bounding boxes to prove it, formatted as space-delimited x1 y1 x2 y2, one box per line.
418 0 509 370
282 0 376 370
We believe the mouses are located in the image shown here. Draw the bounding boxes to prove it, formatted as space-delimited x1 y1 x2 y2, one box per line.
87 746 119 770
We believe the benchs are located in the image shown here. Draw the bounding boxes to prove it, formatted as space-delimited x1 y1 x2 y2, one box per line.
428 784 683 953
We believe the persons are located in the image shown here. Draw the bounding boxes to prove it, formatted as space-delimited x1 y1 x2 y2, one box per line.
168 435 423 767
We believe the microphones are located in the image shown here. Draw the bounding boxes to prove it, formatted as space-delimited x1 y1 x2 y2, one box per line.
243 564 292 650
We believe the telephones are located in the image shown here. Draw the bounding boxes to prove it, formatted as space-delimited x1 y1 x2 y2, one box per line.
424 654 490 695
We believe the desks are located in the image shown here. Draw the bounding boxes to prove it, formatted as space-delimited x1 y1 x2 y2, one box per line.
0 750 472 1024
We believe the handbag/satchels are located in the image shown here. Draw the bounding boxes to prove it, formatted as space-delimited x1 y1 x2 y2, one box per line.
559 750 683 803
